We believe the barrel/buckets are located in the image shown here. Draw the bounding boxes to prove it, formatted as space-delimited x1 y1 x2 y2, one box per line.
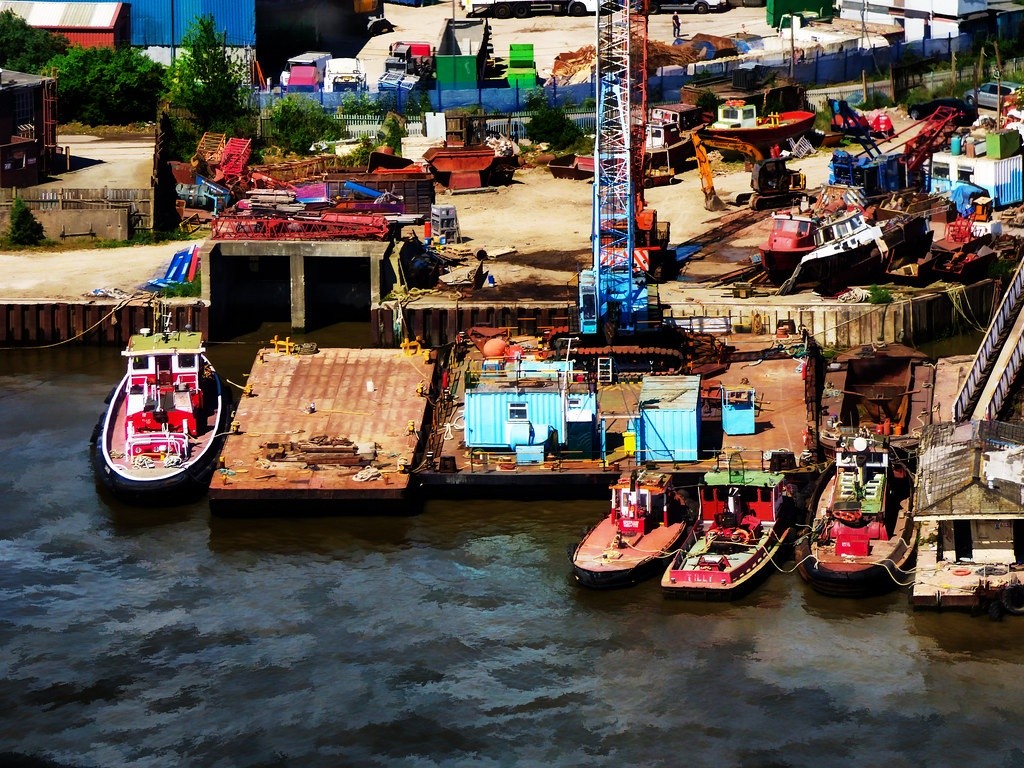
951 136 961 155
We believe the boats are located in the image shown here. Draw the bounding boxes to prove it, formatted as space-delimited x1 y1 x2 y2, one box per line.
659 447 797 589
87 302 224 506
703 97 817 155
571 467 690 585
794 432 918 599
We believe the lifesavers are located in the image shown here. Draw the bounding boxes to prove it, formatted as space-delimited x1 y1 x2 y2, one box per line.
732 529 749 546
832 510 855 522
1002 584 1024 614
952 568 972 577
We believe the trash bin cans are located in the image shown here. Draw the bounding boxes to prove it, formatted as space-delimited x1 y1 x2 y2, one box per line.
622 432 637 456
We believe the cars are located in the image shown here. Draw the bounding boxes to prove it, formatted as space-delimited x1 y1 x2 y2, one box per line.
963 80 1024 110
908 95 979 126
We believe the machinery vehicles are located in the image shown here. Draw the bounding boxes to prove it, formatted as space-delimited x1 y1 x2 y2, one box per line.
547 0 685 378
688 133 811 212
